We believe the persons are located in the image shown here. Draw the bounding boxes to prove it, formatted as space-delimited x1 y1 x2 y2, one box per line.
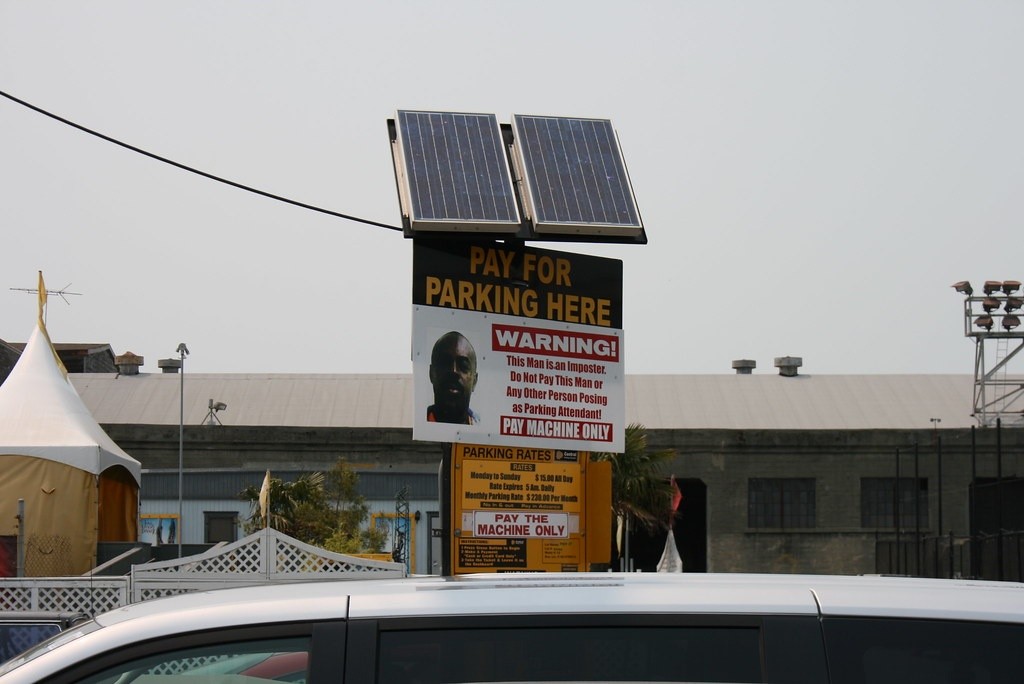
427 331 481 425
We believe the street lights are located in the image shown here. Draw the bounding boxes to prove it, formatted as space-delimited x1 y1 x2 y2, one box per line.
176 343 190 560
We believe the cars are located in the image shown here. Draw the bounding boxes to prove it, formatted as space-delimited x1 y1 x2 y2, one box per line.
0 608 307 684
0 568 1024 684
